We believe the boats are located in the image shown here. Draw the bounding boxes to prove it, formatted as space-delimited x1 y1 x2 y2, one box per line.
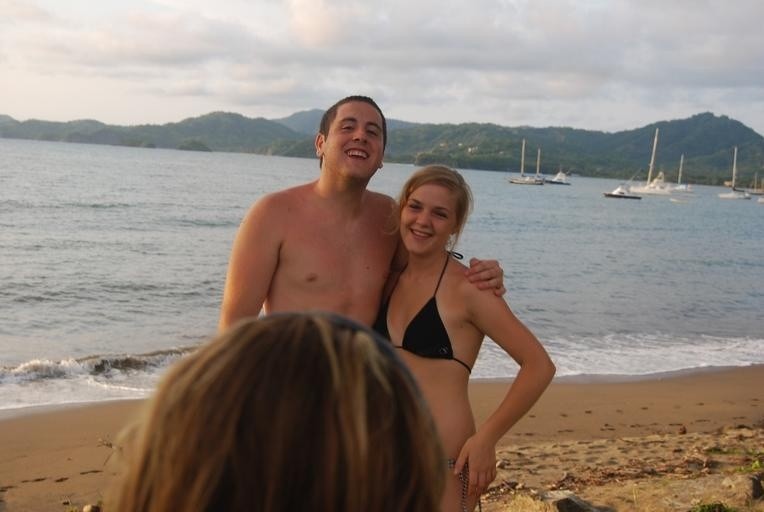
602 167 642 199
544 169 572 186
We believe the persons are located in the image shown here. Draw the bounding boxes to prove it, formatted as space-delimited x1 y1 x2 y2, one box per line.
376 166 555 512
114 313 441 511
215 94 507 333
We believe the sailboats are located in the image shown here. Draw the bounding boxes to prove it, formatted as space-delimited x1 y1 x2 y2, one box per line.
668 152 692 191
521 147 548 180
505 137 544 185
628 128 671 194
717 146 764 205
